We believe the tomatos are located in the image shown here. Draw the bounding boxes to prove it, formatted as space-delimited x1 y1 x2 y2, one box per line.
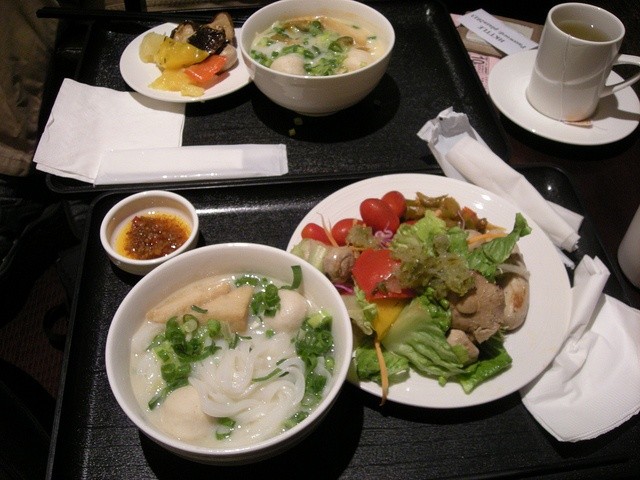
301 224 330 245
360 198 399 232
332 220 362 247
383 191 405 220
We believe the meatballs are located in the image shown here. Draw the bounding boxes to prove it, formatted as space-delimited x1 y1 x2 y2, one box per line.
217 43 239 72
163 387 206 439
263 287 309 332
271 54 305 75
342 47 371 70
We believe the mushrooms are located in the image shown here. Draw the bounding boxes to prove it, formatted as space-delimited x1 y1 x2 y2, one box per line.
208 12 235 44
323 249 356 283
497 271 529 331
187 24 226 53
171 13 198 43
451 272 506 343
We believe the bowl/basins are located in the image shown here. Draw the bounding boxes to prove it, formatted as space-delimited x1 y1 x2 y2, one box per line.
239 0 396 118
104 242 352 468
98 190 201 274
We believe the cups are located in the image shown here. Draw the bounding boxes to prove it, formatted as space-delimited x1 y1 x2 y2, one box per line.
618 205 639 286
525 2 640 122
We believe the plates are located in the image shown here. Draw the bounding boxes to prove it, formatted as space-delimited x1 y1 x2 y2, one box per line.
119 22 252 105
285 170 571 409
487 51 640 146
